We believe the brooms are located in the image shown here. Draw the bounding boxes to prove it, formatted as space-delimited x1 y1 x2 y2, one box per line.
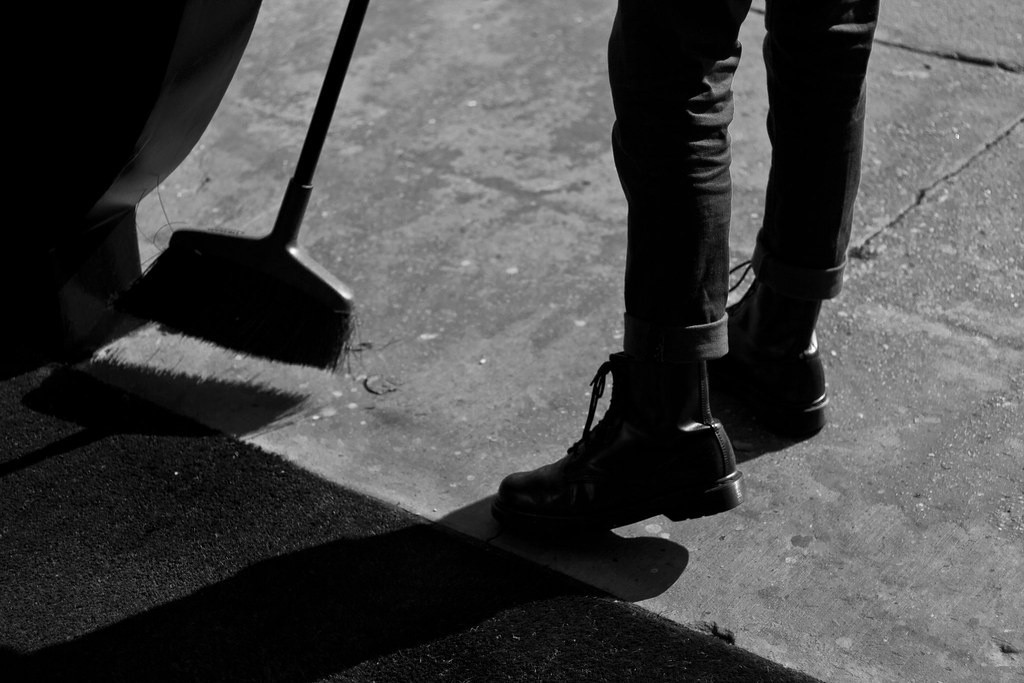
109 0 373 373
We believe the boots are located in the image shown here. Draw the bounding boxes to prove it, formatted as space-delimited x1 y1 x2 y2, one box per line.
704 262 832 438
489 349 747 542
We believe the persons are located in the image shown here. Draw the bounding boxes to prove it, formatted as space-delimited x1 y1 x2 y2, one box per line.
492 1 882 531
